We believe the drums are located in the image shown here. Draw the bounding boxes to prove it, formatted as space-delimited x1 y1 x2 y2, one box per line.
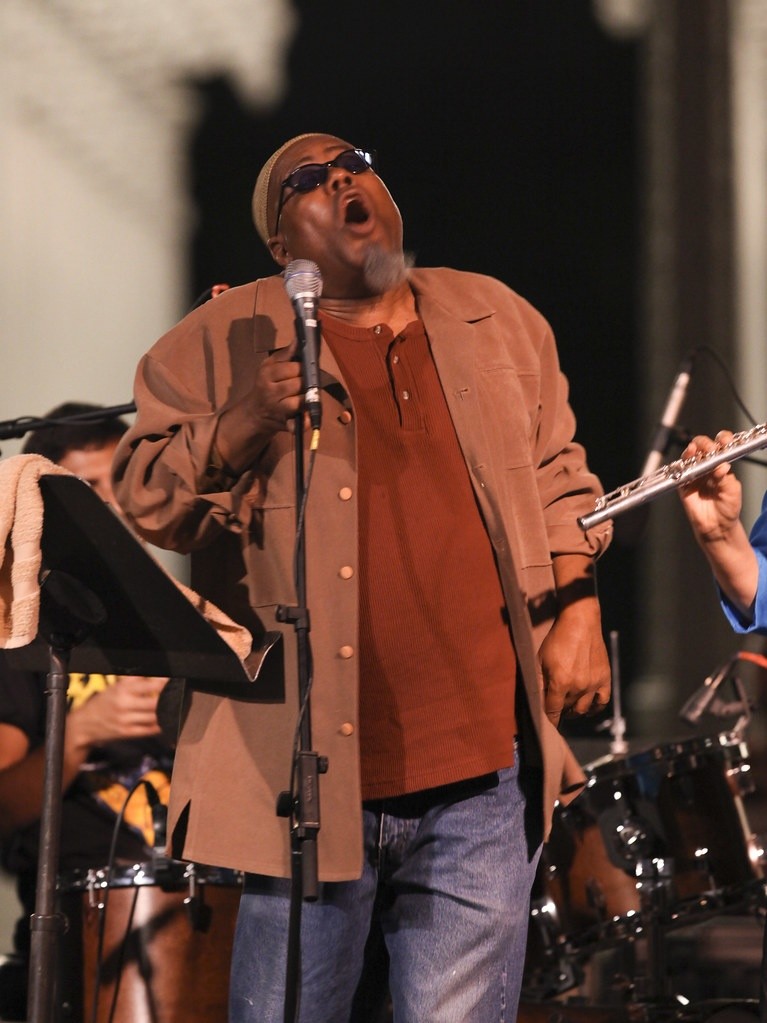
51 855 244 1020
542 726 767 946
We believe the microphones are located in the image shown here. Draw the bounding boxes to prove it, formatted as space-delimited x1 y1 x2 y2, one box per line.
282 259 322 431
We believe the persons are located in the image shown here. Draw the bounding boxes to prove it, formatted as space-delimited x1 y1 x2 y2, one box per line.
679 431 767 633
0 402 173 1019
110 132 610 1022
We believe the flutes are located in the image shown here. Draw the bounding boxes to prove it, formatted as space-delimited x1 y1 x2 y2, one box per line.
577 422 766 532
